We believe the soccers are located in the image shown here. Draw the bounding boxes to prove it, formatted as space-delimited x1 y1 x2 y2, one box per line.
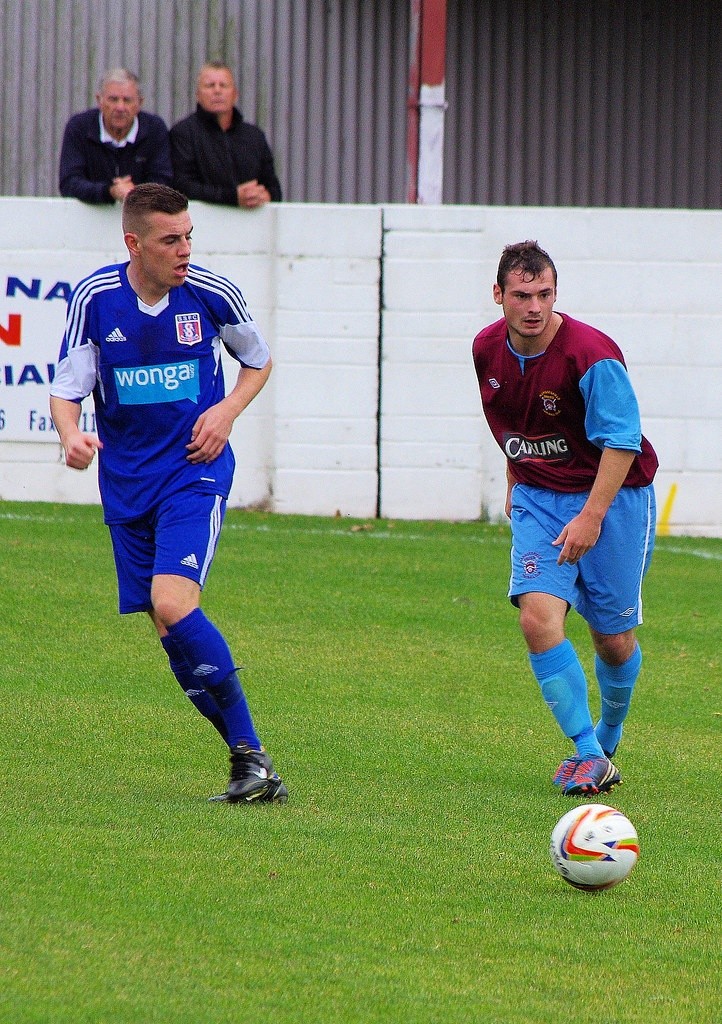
549 803 640 893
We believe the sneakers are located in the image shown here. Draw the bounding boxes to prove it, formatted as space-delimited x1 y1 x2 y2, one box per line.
564 755 623 798
208 776 288 803
226 739 273 796
553 723 621 786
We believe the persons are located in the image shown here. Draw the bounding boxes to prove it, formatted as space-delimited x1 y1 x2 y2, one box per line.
472 240 659 798
49 182 288 804
170 60 283 208
58 67 174 205
